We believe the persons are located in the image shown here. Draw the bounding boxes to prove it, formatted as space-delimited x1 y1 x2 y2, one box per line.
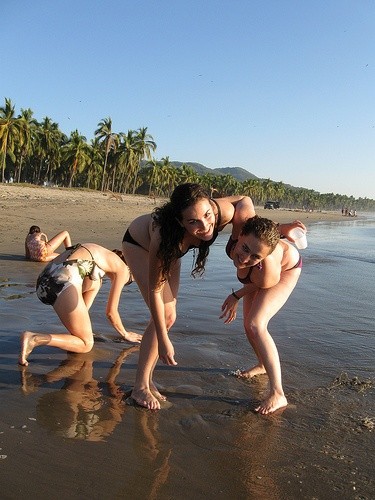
211 196 303 416
123 183 306 410
25 225 73 260
20 242 144 366
341 207 355 218
